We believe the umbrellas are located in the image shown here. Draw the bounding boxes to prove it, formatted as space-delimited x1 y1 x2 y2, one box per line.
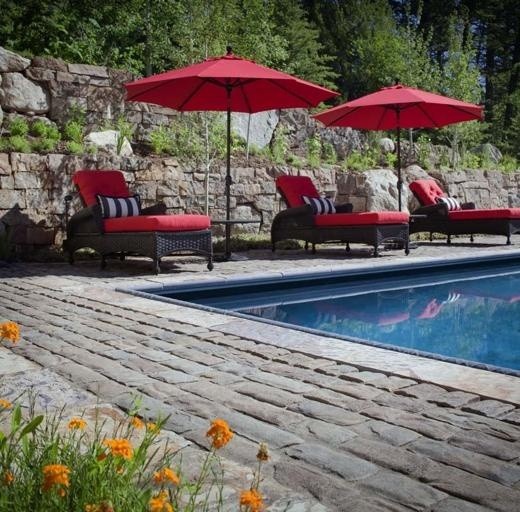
120 46 342 262
309 77 486 250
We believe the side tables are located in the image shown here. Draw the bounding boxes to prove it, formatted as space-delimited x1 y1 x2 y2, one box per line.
213 220 262 262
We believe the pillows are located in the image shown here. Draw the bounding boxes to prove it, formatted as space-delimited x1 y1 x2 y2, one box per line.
95 195 141 218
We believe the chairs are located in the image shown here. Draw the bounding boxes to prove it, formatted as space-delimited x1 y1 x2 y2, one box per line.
273 176 520 257
61 170 213 276
